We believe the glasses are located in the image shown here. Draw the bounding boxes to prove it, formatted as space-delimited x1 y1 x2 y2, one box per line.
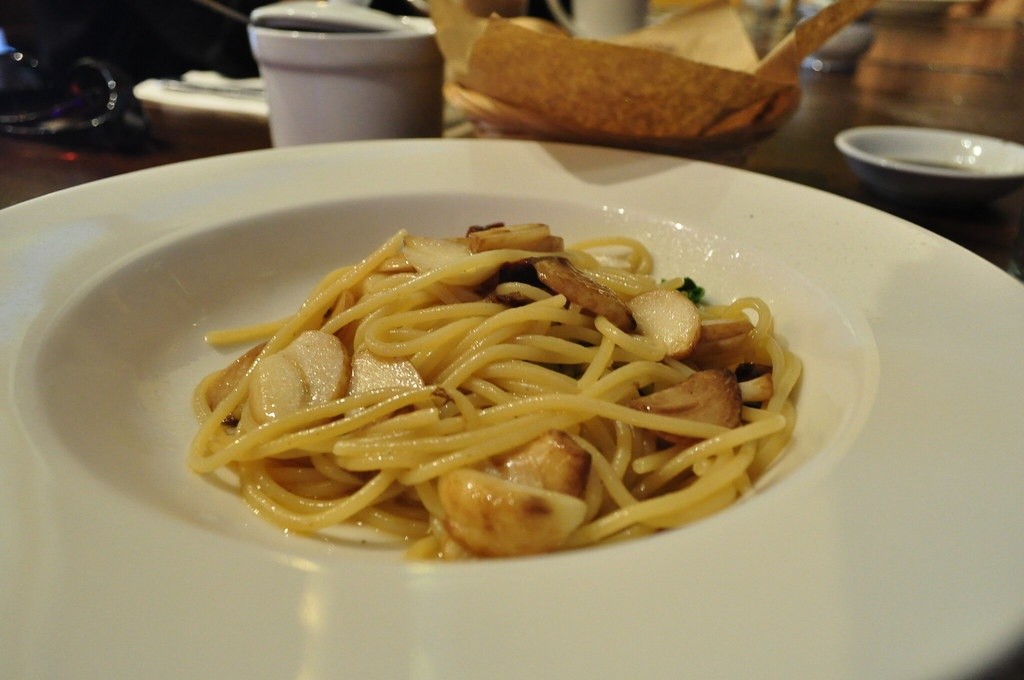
0 55 171 156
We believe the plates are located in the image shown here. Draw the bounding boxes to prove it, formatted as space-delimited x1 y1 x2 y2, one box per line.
0 122 1023 680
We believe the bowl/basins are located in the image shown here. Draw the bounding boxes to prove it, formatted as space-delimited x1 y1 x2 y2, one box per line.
834 126 1024 209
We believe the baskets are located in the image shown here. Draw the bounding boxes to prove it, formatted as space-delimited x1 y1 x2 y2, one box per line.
442 76 801 170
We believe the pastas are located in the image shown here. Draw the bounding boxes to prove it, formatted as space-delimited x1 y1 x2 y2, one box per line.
189 228 803 556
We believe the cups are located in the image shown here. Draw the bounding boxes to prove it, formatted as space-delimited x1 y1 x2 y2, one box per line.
546 0 649 42
246 0 446 154
